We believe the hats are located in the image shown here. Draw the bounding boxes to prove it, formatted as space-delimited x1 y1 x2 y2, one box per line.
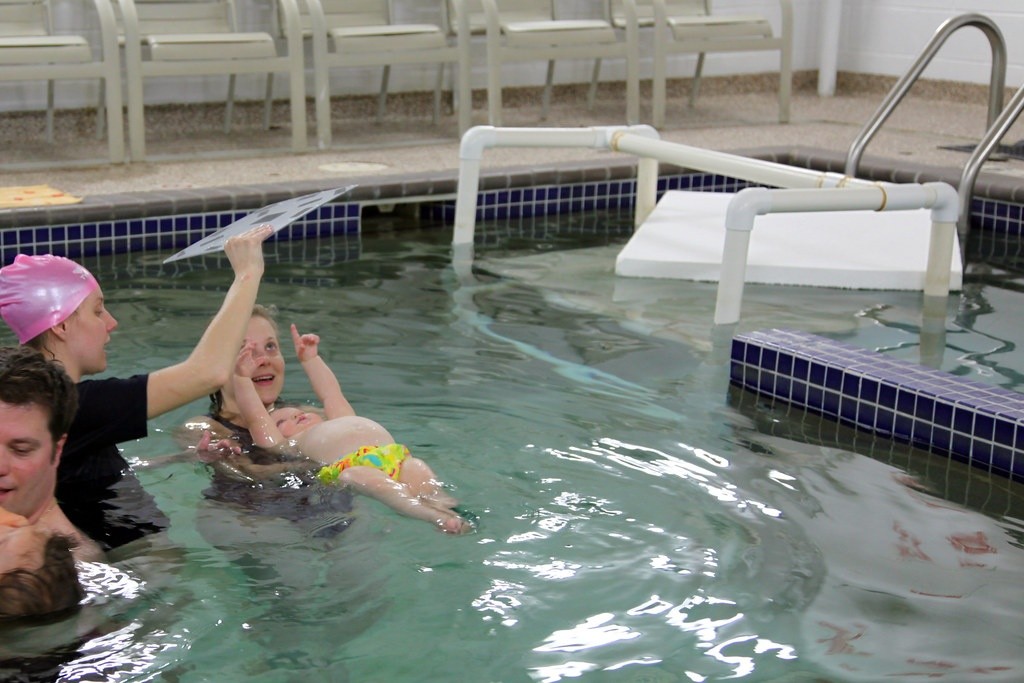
0 253 98 344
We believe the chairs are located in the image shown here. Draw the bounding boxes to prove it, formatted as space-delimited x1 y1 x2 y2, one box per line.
432 0 640 126
0 0 126 167
263 0 473 140
97 0 307 162
586 0 794 129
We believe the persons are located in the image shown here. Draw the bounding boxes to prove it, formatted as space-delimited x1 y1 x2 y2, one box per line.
0 223 276 492
0 523 84 622
178 301 326 456
231 323 474 536
0 344 105 561
